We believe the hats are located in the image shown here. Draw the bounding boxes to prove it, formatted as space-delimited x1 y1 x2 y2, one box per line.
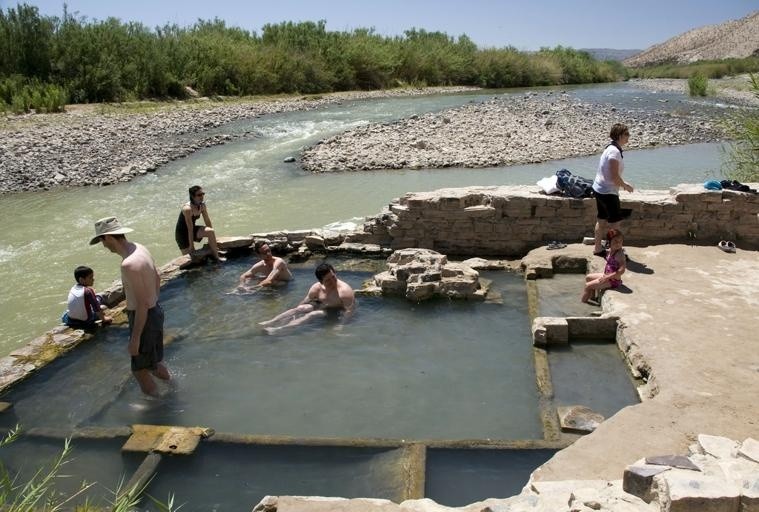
89 216 134 244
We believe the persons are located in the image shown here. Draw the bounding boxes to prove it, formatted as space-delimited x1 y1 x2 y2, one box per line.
257 262 358 337
590 122 636 263
87 217 172 416
175 185 228 266
580 227 628 304
63 266 112 333
226 238 296 298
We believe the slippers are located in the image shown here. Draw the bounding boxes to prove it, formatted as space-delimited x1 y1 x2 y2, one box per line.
718 240 736 252
548 241 567 249
594 250 606 255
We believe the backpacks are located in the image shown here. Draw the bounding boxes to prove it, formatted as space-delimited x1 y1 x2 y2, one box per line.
556 168 596 198
